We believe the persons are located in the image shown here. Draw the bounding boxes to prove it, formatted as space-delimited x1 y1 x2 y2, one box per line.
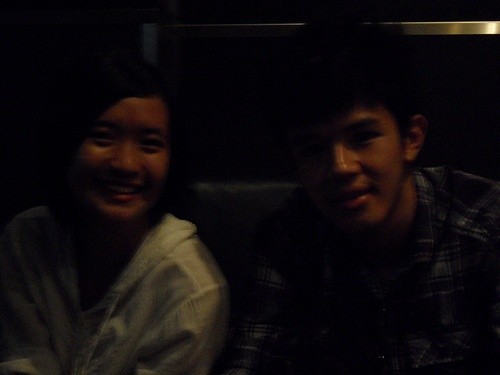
207 37 500 375
0 51 231 375
